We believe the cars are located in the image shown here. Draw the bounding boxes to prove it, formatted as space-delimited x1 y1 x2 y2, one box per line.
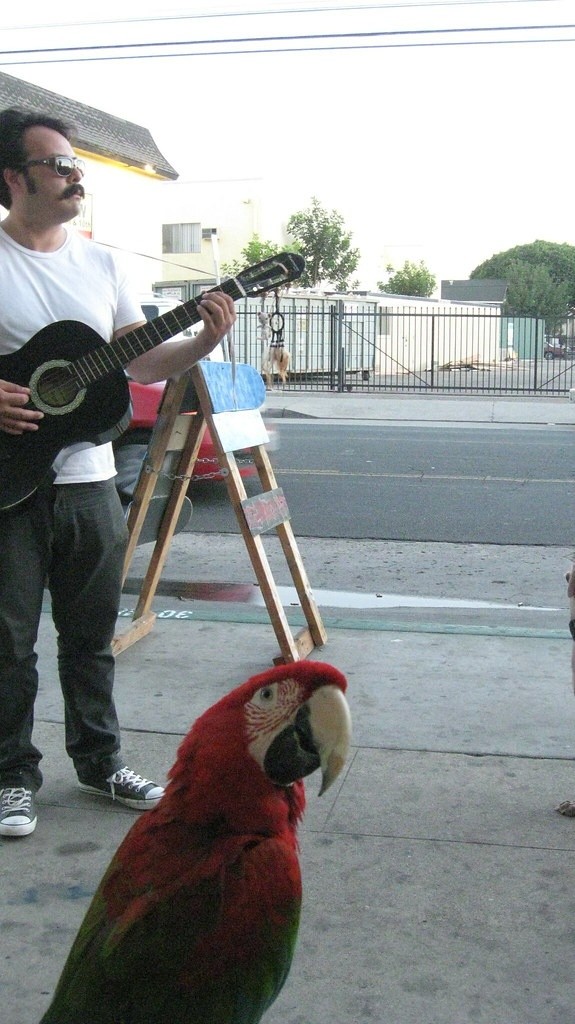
544 341 568 361
114 369 279 506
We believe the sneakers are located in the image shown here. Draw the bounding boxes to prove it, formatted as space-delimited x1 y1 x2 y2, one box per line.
0 782 37 836
77 764 166 810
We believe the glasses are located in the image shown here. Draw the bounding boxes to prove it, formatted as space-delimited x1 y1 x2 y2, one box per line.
11 155 84 179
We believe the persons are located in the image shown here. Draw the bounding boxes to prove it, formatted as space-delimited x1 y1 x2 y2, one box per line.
0 105 239 836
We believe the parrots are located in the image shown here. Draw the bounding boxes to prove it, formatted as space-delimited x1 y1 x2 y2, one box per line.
37 660 356 1024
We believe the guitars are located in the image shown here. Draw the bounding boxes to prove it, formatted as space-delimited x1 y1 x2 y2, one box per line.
0 251 309 511
